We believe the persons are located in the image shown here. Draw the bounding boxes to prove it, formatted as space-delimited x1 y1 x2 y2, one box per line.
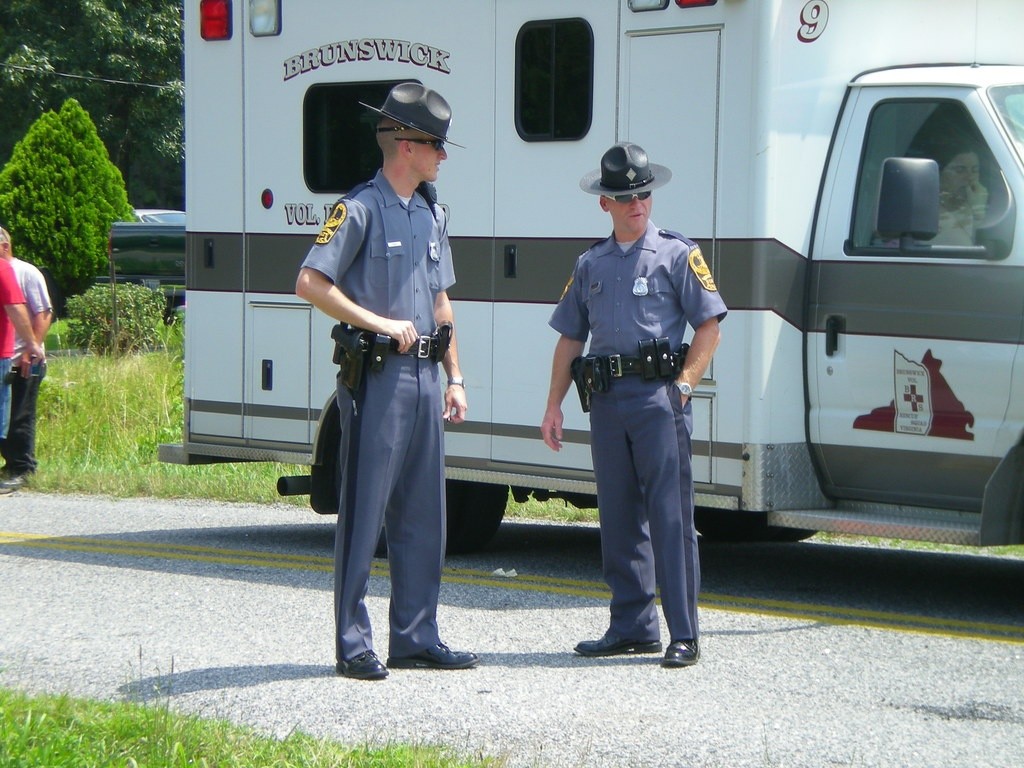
918 141 987 245
544 142 729 667
296 82 476 679
0 228 54 490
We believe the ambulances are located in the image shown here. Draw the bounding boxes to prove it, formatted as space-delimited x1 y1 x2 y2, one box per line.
154 1 1024 553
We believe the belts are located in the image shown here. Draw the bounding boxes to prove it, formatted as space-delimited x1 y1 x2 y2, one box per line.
371 334 437 358
603 354 643 377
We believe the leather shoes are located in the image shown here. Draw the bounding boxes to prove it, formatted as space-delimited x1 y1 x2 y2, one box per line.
336 650 388 678
663 637 701 665
386 643 478 669
573 628 662 656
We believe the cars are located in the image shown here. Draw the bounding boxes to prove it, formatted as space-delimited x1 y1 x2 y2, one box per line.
41 207 185 320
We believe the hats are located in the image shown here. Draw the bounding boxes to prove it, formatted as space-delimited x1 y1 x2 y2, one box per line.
358 83 466 150
579 142 673 196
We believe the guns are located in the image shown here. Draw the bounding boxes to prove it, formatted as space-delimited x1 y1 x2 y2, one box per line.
571 354 593 413
331 325 370 389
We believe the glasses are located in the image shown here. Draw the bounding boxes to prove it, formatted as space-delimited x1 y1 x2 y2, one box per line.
605 191 653 203
394 137 443 151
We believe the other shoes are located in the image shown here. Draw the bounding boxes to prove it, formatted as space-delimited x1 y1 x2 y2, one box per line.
0 477 18 492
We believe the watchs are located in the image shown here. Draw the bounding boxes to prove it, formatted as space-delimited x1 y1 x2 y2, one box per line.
675 381 691 395
448 378 466 389
